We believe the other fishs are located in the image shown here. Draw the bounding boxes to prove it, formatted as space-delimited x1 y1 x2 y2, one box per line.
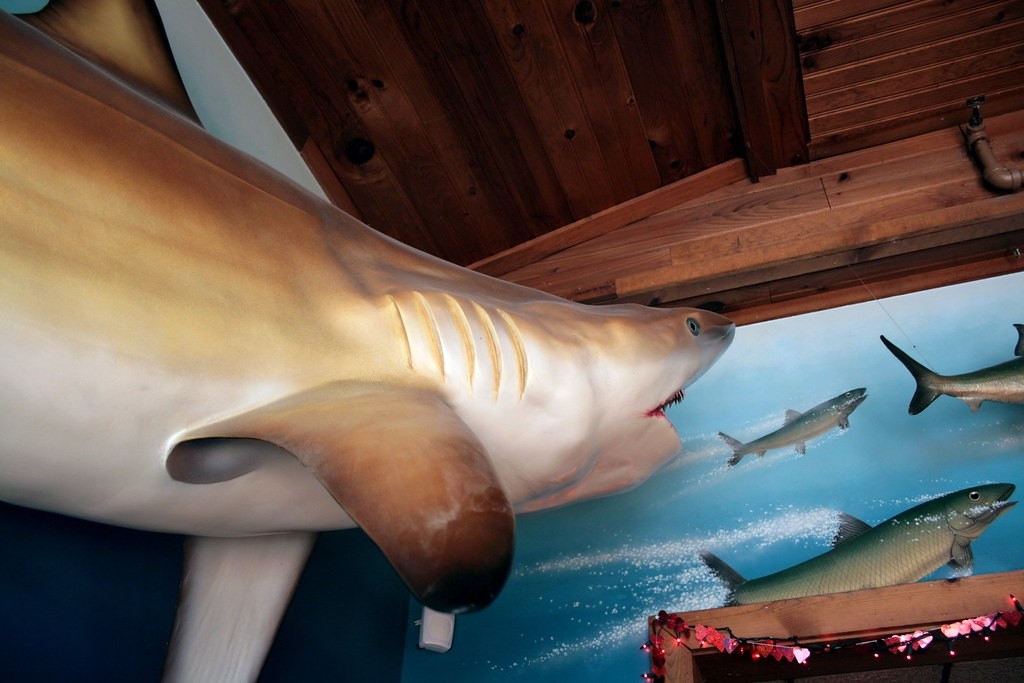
698 483 1019 607
717 387 869 467
881 334 1024 416
